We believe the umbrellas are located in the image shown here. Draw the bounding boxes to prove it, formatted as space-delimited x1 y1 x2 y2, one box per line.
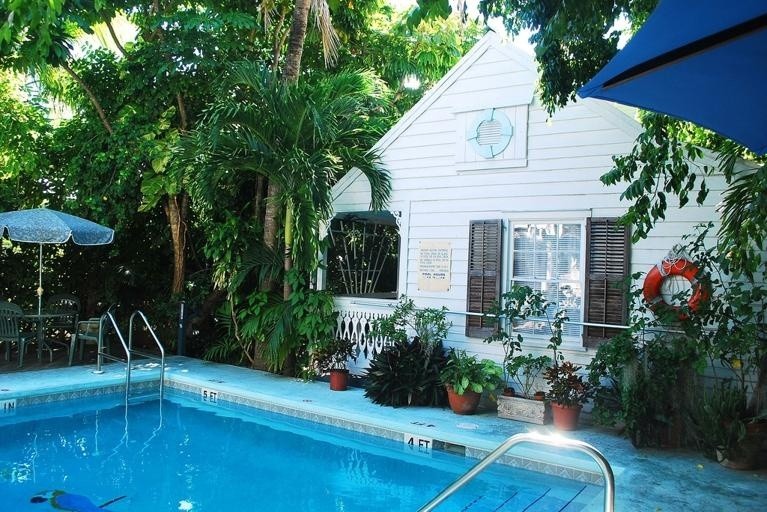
0 208 115 316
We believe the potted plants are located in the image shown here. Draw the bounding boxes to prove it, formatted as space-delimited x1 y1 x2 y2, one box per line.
497 355 552 424
437 347 503 416
328 337 358 390
682 375 767 471
541 359 590 430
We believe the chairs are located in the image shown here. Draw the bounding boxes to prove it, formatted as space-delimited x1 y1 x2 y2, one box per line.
40 293 81 353
69 301 119 367
0 300 36 369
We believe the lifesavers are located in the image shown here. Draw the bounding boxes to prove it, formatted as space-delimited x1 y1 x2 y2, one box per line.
644 260 709 319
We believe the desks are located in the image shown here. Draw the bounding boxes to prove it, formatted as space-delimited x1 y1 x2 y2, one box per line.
4 308 74 362
